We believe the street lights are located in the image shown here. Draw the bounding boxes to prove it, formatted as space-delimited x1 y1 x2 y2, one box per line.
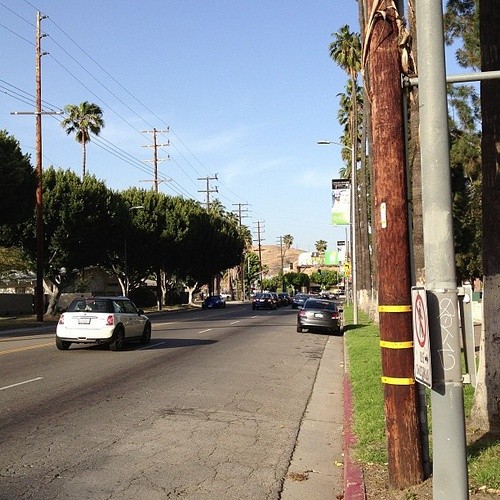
318 140 358 325
123 205 145 278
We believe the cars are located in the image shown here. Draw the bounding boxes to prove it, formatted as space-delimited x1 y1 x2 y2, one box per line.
56 296 151 352
202 294 226 310
252 292 277 311
296 298 343 336
276 284 345 312
